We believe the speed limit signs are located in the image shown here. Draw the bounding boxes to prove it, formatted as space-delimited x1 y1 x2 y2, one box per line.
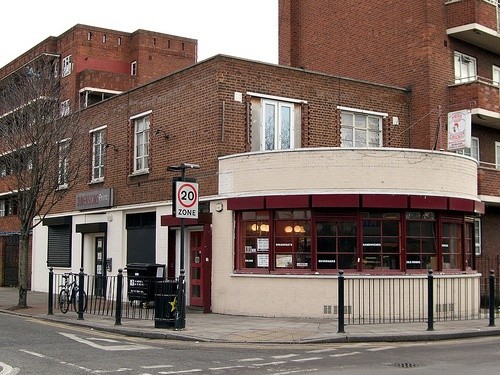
175 181 199 219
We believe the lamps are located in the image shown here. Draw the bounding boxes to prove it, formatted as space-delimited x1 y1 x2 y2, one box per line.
105 143 119 152
156 129 169 140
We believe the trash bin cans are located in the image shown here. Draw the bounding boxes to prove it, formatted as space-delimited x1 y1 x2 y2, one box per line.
125 262 165 308
153 278 186 330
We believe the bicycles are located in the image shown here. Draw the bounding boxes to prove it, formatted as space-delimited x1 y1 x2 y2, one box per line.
58 273 87 314
